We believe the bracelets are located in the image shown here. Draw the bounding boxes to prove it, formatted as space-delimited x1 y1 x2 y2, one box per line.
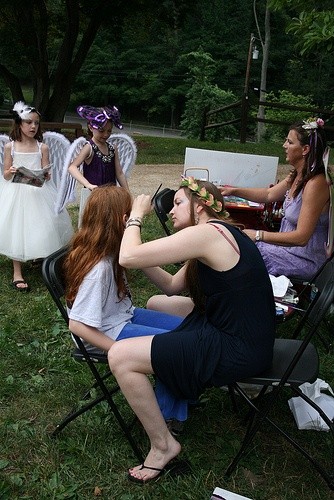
125 216 143 228
255 230 264 241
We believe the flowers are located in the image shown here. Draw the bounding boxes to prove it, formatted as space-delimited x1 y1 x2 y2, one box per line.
302 117 324 136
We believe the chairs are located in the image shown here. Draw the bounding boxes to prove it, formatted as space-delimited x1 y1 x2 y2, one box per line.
41 189 334 492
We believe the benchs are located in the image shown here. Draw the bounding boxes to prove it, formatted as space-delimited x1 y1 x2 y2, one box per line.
0 119 82 139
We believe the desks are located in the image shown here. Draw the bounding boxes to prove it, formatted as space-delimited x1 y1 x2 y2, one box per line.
224 200 266 211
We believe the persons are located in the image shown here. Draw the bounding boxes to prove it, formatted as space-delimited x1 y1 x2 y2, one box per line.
108 176 278 485
67 106 136 234
64 184 188 437
0 101 74 292
217 115 331 278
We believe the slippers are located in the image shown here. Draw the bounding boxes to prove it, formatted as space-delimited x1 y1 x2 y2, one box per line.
10 280 30 290
127 461 188 485
